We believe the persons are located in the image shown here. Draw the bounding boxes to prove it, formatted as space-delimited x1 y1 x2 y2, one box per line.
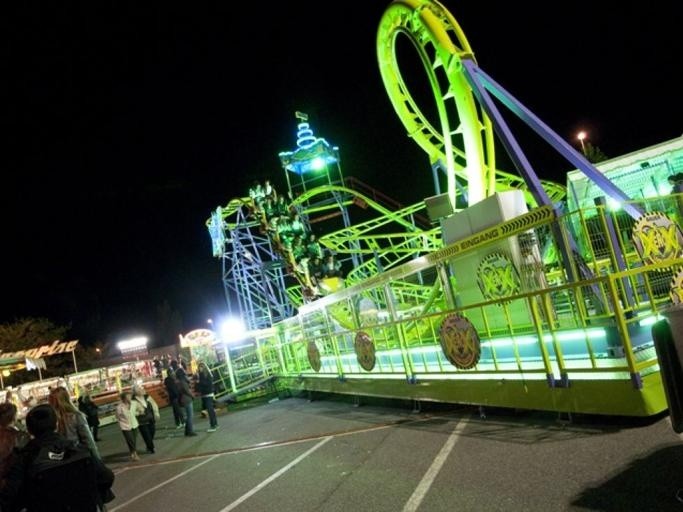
135 372 142 384
0 403 30 461
131 384 160 454
675 172 682 181
163 366 186 429
193 363 220 433
83 396 104 443
50 389 101 462
76 394 84 411
4 387 38 430
666 175 682 193
119 368 130 383
173 368 198 437
248 176 343 296
0 403 114 512
115 392 145 463
152 353 188 379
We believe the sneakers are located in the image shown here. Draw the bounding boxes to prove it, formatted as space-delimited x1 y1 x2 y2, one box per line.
185 432 197 436
205 425 220 432
176 422 186 429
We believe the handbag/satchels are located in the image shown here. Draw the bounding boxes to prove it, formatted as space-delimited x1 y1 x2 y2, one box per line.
137 409 154 421
